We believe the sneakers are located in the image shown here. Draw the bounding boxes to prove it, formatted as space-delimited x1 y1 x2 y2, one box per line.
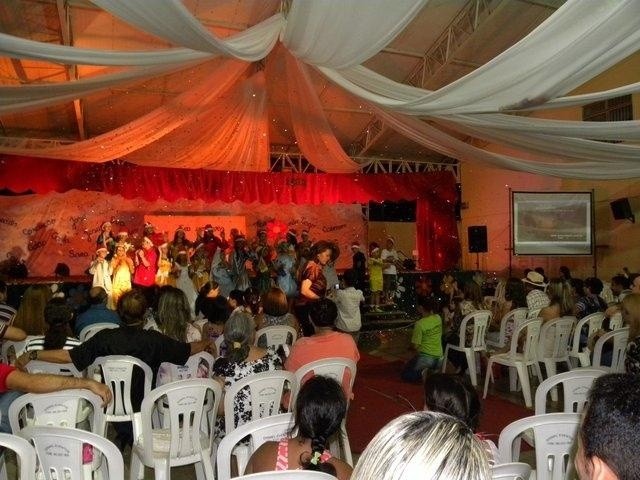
369 304 375 313
375 305 384 313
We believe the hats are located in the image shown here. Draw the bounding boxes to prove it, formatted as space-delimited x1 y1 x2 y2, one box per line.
256 246 269 272
522 272 547 287
176 224 186 233
274 238 286 246
97 245 109 255
256 229 267 234
144 233 157 247
205 224 212 231
157 239 168 249
289 228 297 235
302 229 309 235
178 245 186 254
119 226 128 236
387 236 395 243
352 242 359 248
235 235 244 241
370 243 378 254
114 242 126 257
144 222 151 228
100 221 111 231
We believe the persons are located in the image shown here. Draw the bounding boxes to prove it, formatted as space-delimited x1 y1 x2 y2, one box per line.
229 235 251 290
147 288 215 409
559 266 571 280
350 410 490 480
110 242 134 310
610 274 630 302
297 229 314 267
28 351 39 361
97 221 114 257
294 239 340 336
89 245 112 309
15 282 52 337
622 292 640 374
167 226 202 259
155 243 175 287
203 224 227 264
488 278 574 354
285 229 298 260
170 246 199 319
366 242 384 313
143 222 154 237
275 239 297 296
381 236 399 305
21 297 89 414
423 376 485 441
254 287 298 348
194 281 231 326
351 241 366 275
588 276 640 368
401 292 444 382
132 233 164 287
0 282 16 325
244 375 353 480
575 278 607 346
520 271 551 311
0 362 113 408
327 268 366 345
442 279 491 376
0 317 28 343
227 289 244 312
12 290 212 453
255 245 274 292
103 227 138 257
74 285 124 337
574 374 640 480
252 230 270 251
281 299 360 411
568 278 585 302
210 247 236 296
209 313 291 444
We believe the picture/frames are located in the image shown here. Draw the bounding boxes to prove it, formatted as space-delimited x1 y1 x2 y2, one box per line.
512 191 595 257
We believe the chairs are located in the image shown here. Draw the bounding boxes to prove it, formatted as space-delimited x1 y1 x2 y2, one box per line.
609 311 624 331
254 325 297 349
512 369 610 470
8 389 108 480
293 358 356 468
130 379 222 480
599 284 614 303
485 308 530 348
492 463 530 480
79 323 119 344
572 327 631 376
442 310 494 390
213 335 225 356
156 351 214 429
86 355 153 440
1 335 39 365
482 318 543 409
0 432 35 479
484 282 503 320
566 312 607 368
527 309 543 320
15 425 125 479
498 413 583 480
22 361 93 431
517 315 578 402
214 371 297 477
217 412 340 480
231 470 338 480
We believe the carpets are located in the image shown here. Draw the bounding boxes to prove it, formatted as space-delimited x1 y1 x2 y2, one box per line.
339 349 534 452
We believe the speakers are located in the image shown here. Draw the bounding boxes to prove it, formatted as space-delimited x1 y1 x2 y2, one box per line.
467 225 489 254
610 197 632 220
